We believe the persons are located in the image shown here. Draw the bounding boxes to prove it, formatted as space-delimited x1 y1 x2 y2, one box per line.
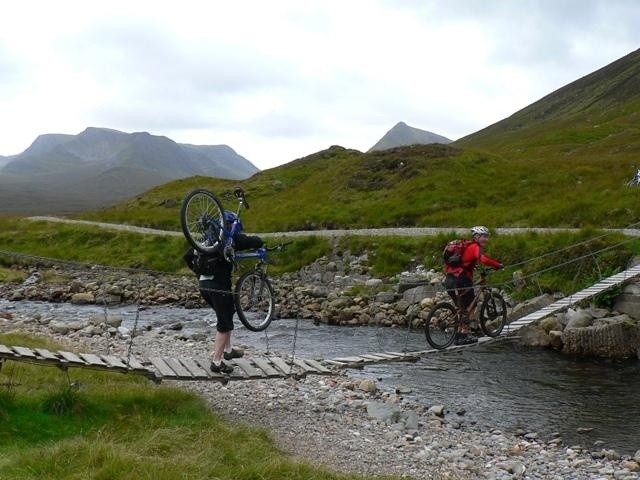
183 209 264 373
443 225 504 346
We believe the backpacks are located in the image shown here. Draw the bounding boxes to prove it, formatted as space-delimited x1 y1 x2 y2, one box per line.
193 235 222 274
442 239 472 266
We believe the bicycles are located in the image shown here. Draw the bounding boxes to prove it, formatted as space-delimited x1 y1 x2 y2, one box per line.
179 183 295 330
425 255 507 351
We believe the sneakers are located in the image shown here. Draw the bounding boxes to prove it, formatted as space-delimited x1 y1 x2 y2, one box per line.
223 347 244 360
455 332 478 345
210 361 234 373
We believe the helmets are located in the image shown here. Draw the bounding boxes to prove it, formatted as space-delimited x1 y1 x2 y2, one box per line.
470 226 489 235
225 209 238 221
